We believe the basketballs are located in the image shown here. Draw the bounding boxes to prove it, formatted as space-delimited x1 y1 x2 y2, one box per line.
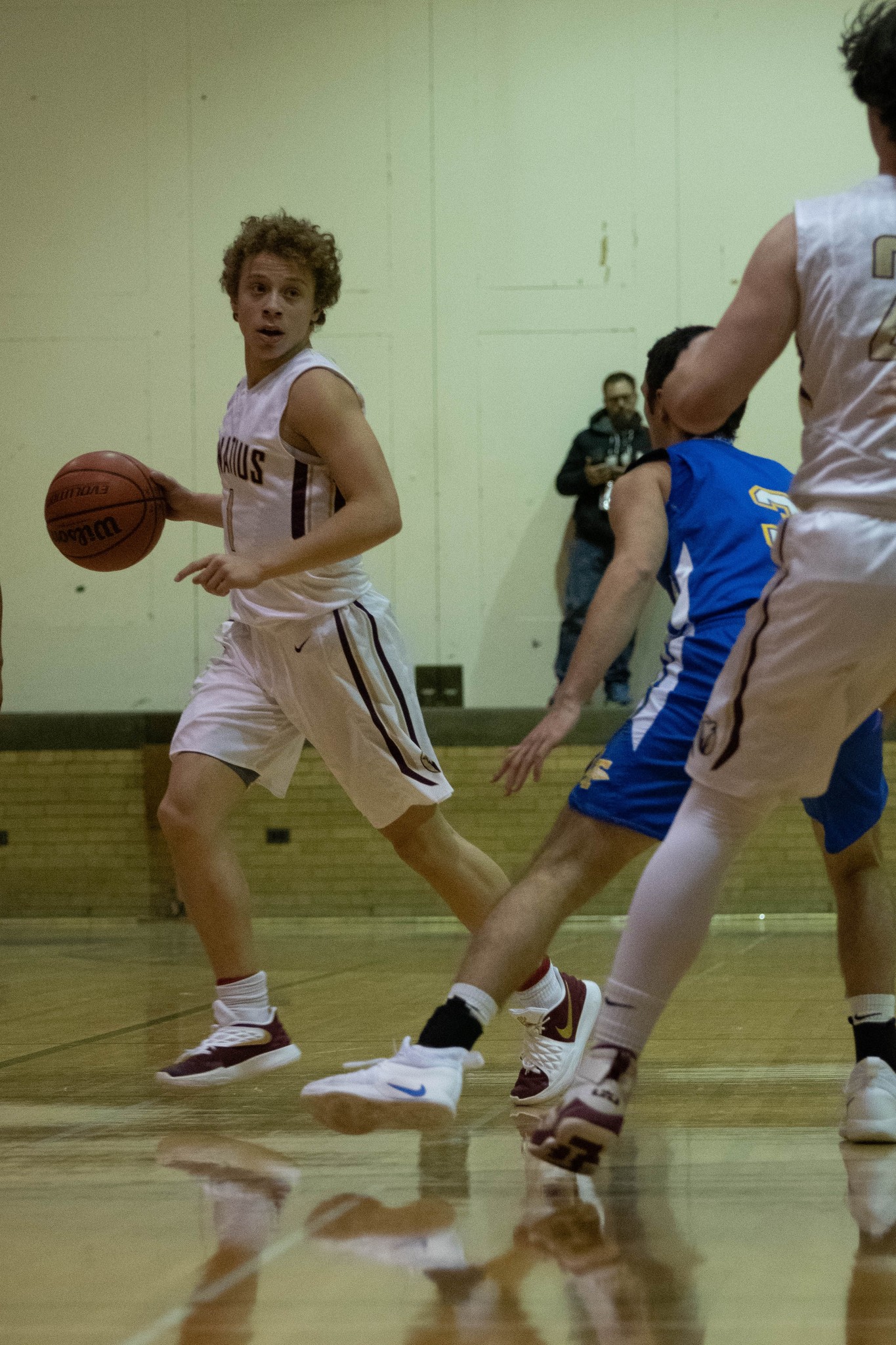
44 450 167 573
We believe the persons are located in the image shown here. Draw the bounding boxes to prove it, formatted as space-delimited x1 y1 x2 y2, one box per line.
299 323 896 1151
142 208 602 1107
549 371 659 710
520 0 896 1174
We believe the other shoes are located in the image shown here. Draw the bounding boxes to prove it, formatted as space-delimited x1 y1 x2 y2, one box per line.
604 685 637 711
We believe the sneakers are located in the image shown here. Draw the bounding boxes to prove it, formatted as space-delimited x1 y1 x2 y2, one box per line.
157 1000 302 1090
520 1044 638 1175
836 1057 896 1145
297 1035 483 1136
301 1191 461 1265
834 1143 896 1235
507 966 601 1107
511 1161 620 1269
155 1132 300 1207
